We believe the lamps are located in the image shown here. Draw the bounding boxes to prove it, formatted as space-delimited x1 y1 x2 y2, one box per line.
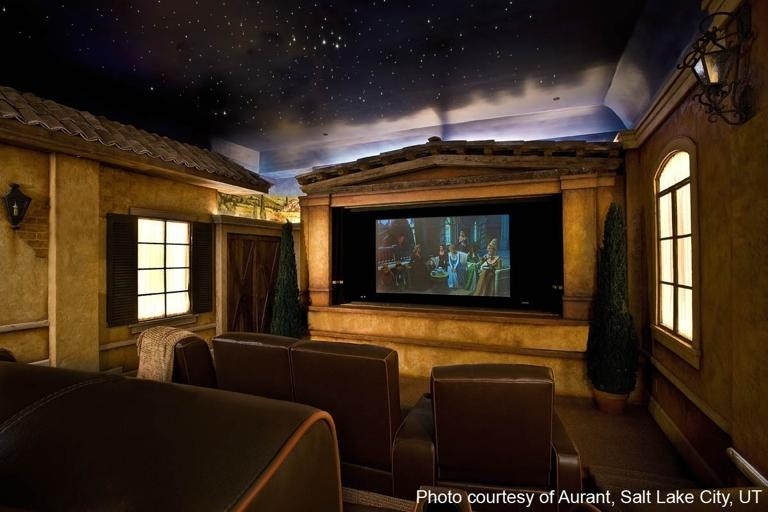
1 182 32 230
677 10 759 126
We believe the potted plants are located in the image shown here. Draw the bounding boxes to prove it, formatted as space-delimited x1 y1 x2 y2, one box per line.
584 201 640 415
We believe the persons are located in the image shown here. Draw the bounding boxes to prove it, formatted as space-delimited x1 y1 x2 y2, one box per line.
379 231 504 294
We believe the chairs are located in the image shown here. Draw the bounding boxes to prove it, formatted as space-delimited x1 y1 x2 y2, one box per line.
0 327 583 512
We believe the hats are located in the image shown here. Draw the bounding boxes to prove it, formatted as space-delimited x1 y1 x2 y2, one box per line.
487 238 497 250
